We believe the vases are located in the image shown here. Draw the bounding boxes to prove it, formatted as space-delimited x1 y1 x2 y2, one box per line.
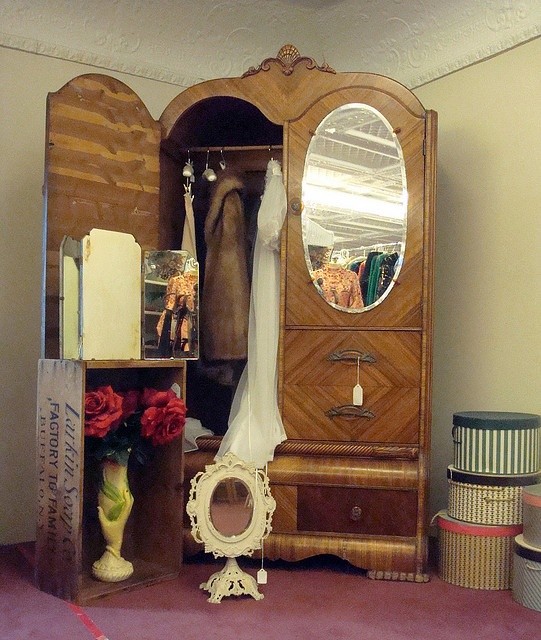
93 447 137 582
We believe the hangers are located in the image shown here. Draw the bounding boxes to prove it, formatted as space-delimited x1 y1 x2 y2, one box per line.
339 241 403 259
183 147 196 183
201 146 219 183
218 147 227 171
268 145 277 163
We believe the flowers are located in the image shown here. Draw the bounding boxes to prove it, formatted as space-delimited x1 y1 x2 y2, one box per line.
81 383 191 470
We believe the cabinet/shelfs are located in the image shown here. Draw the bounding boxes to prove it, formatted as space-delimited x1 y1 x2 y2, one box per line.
38 42 442 585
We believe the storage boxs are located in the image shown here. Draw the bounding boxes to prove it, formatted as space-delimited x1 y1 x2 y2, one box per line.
429 507 527 592
36 359 188 607
520 482 541 552
509 534 541 614
448 409 541 477
445 461 541 527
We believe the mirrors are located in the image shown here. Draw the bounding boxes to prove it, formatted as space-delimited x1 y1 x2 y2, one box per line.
299 102 411 315
185 451 278 606
143 250 203 360
